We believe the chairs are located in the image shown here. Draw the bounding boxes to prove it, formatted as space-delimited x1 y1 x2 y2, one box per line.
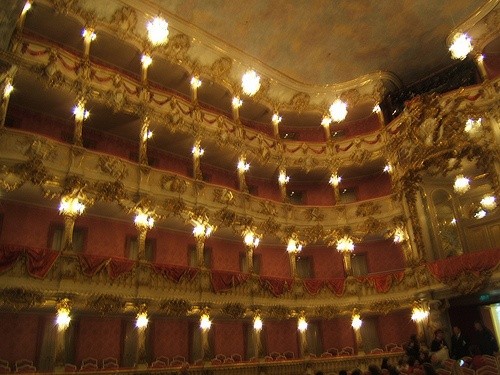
1 340 500 375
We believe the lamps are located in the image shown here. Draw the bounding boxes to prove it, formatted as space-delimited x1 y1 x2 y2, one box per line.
0 0 500 335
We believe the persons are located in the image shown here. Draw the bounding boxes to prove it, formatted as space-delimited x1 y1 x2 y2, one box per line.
449 324 472 361
313 321 451 375
472 322 499 357
